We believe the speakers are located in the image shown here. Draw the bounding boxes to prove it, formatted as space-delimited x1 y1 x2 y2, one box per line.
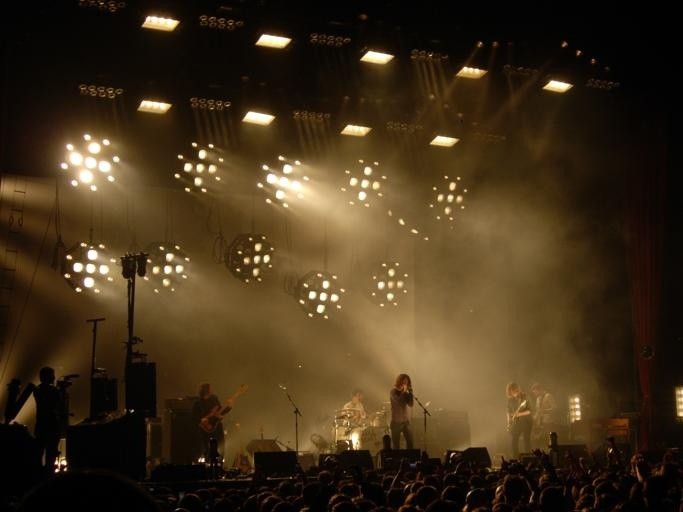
319 449 374 475
520 454 551 471
548 443 590 466
443 446 493 471
67 361 211 484
381 448 420 472
245 437 297 477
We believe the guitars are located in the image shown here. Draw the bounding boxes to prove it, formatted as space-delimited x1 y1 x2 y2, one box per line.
505 400 527 432
202 384 248 432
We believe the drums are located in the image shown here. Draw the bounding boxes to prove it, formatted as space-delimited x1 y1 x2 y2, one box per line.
335 409 362 428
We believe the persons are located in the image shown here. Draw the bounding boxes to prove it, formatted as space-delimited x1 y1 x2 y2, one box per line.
341 388 368 426
189 382 234 465
504 381 534 459
529 383 559 451
31 365 69 475
2 419 683 512
390 373 415 450
